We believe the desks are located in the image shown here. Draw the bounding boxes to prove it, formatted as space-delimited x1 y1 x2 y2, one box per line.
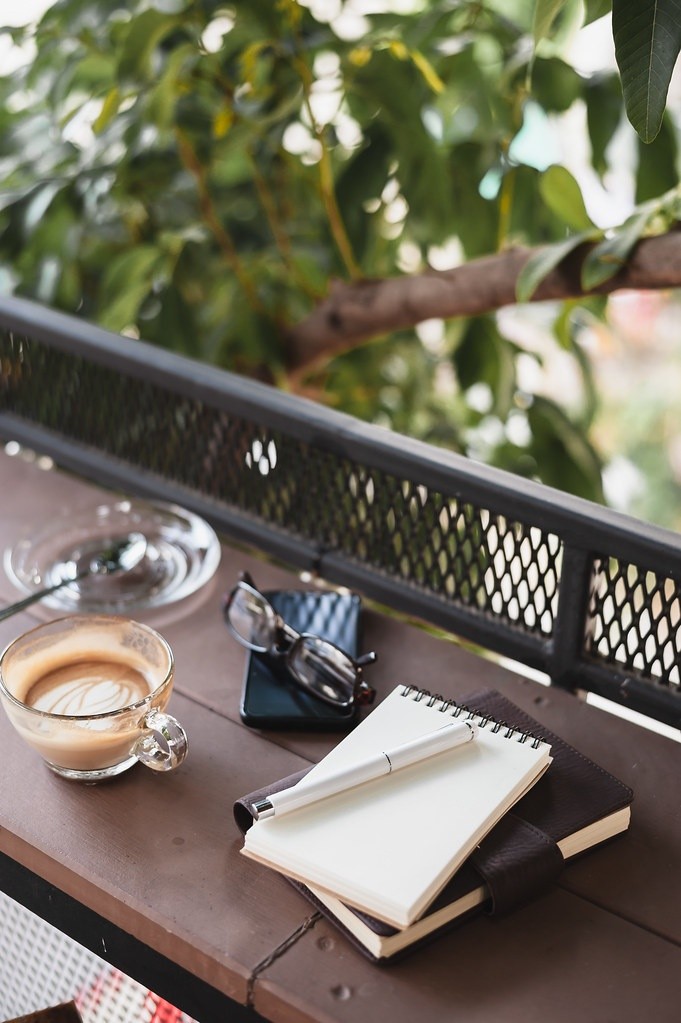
0 447 681 1022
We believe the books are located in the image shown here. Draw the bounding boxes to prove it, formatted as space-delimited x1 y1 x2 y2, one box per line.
233 685 635 968
240 688 555 932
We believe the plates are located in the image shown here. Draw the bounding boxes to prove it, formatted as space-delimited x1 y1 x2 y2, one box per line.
3 494 222 611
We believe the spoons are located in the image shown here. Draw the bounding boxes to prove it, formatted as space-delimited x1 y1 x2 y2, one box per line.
0 532 147 623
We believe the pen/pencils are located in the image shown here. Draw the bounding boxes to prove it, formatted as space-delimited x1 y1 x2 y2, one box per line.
250 719 475 821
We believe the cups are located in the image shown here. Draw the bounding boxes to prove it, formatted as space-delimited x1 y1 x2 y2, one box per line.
0 612 189 781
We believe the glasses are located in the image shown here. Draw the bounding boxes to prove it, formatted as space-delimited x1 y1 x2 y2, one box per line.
222 570 377 707
